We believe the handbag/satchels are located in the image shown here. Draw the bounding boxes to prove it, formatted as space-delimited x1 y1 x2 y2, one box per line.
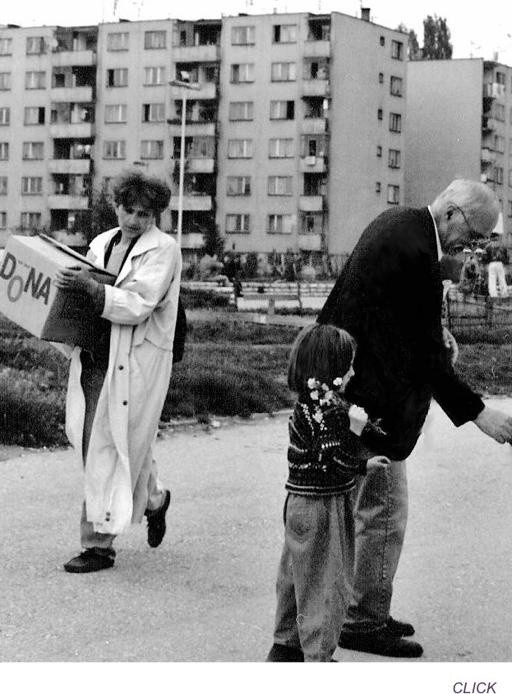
170 294 187 364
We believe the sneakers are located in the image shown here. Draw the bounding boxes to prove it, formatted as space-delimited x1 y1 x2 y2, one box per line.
338 621 424 659
387 616 416 636
64 546 117 573
266 643 338 661
144 488 171 548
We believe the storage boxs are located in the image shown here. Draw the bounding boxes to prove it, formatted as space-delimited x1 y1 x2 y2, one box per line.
1 232 116 345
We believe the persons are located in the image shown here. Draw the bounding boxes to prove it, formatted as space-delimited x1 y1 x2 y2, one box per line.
485 232 509 304
51 172 187 572
264 320 392 660
459 247 485 294
318 177 511 656
